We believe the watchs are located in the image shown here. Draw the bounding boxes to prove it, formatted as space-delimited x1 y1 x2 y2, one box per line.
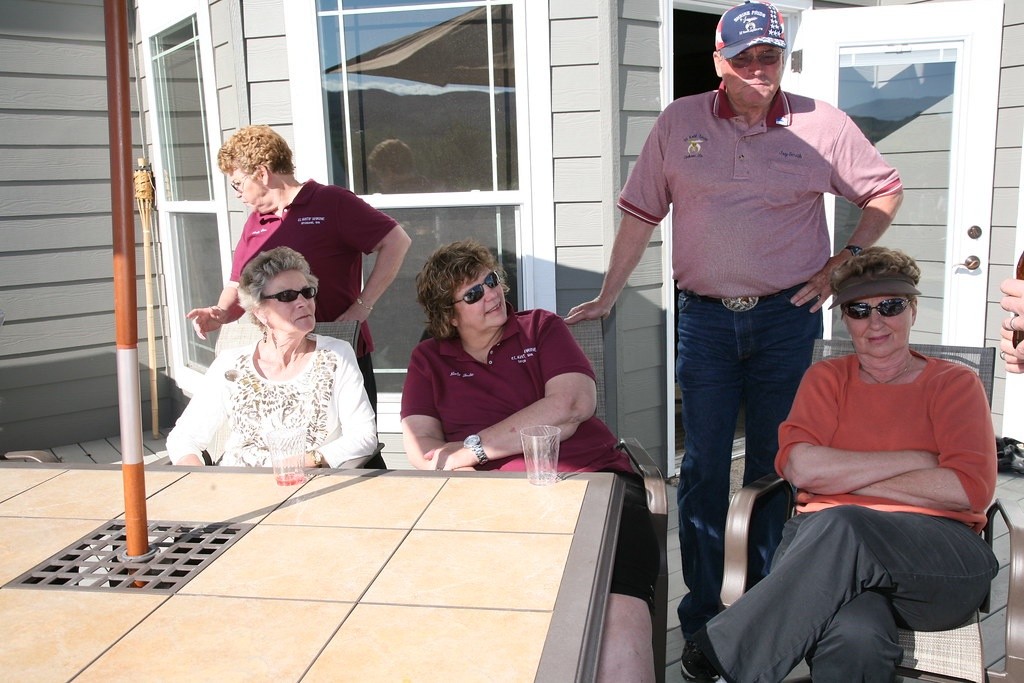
845 246 862 255
463 434 488 464
309 450 322 465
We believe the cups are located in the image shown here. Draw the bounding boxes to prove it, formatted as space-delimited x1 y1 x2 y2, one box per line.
520 426 561 487
265 422 306 485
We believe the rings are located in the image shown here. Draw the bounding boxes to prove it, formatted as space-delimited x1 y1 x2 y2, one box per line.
1000 351 1005 360
1010 317 1017 330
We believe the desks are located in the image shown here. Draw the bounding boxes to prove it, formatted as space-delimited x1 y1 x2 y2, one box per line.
0 461 627 683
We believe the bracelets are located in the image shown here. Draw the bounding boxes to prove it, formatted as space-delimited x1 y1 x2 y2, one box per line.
357 298 374 310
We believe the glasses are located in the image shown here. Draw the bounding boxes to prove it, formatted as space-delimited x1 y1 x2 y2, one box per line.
726 49 784 68
447 270 501 307
261 286 317 302
231 170 256 192
842 296 912 319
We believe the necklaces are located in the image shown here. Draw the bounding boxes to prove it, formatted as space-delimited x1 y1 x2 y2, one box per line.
860 355 915 383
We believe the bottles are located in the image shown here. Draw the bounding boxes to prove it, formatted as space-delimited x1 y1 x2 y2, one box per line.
1013 250 1024 351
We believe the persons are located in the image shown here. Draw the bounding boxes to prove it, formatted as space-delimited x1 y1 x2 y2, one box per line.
369 139 439 196
558 0 903 683
400 235 659 683
187 126 413 469
998 276 1024 374
697 242 999 683
165 244 377 471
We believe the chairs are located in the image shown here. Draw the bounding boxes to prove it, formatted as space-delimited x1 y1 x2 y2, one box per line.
720 338 1024 683
557 303 668 683
214 320 386 468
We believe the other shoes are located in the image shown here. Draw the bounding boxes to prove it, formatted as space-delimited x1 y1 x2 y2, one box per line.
681 640 722 683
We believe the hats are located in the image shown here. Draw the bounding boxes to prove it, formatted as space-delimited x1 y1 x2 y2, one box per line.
826 271 922 310
714 0 787 59
